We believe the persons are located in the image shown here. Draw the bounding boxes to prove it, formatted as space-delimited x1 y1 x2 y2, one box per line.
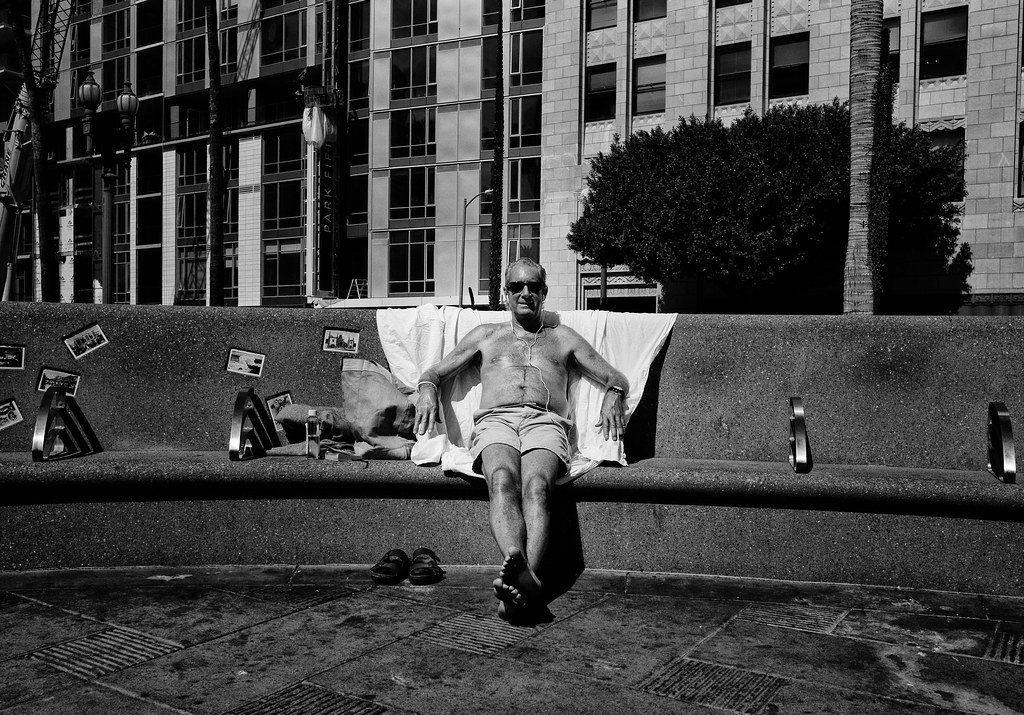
8 412 17 420
413 256 630 620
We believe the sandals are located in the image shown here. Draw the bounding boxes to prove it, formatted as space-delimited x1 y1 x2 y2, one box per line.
369 548 411 586
409 548 443 584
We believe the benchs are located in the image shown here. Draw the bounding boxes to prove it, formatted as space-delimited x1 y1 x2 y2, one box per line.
0 303 1024 598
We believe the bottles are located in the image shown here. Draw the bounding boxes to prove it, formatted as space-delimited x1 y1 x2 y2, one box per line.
305 409 320 459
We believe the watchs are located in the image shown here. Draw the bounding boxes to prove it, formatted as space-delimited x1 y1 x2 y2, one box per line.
606 385 627 402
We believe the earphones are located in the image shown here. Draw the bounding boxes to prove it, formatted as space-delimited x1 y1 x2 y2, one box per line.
542 294 545 301
506 295 510 303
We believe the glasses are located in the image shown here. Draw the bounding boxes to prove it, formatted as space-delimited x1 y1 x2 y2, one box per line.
507 281 544 294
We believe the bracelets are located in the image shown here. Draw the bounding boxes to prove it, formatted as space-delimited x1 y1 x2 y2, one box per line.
417 380 438 394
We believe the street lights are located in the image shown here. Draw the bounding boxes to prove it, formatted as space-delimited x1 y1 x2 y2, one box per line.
458 187 495 307
77 69 141 304
